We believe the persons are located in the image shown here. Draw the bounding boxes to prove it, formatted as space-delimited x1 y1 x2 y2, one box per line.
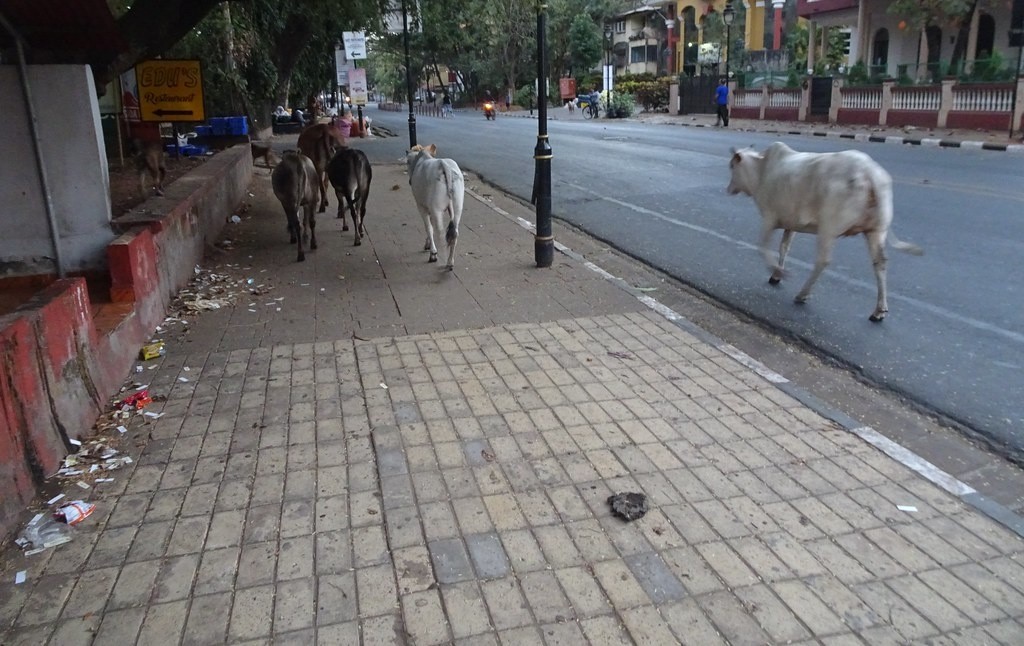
442 89 510 121
713 78 730 127
589 87 600 117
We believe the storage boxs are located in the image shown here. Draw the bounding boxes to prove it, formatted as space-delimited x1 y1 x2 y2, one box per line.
166 116 248 157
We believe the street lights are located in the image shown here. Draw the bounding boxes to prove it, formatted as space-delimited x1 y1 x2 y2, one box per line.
721 2 736 88
527 53 534 116
604 24 613 111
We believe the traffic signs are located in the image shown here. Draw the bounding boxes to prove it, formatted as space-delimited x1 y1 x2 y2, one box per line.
342 30 367 61
133 56 207 125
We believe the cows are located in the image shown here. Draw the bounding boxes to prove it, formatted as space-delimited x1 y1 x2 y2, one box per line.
271 122 372 262
724 141 926 322
404 144 465 273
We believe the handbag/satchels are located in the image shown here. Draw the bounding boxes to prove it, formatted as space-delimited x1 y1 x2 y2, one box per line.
177 132 197 147
726 109 728 116
588 100 592 106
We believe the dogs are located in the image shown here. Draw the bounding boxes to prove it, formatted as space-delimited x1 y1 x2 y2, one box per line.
250 142 278 175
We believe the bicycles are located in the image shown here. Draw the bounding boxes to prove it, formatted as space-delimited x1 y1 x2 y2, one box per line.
582 98 598 119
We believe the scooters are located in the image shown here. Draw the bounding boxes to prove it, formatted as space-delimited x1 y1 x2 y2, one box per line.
482 99 497 121
